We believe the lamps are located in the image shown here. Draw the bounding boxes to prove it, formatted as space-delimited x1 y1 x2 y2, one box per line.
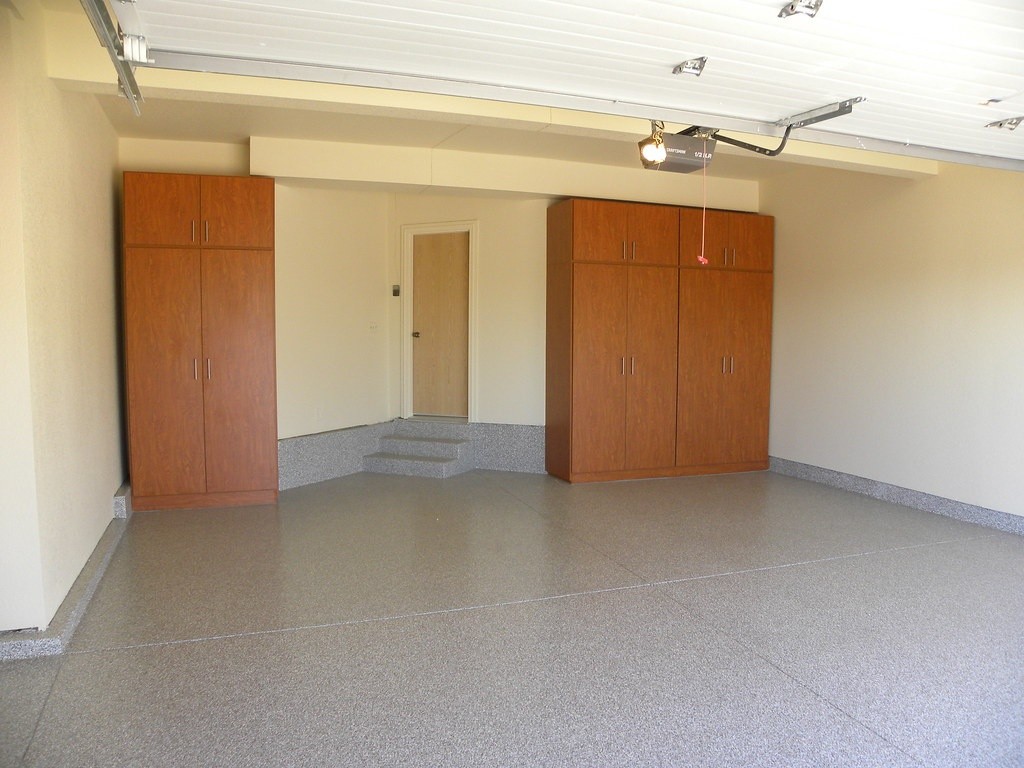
635 95 868 175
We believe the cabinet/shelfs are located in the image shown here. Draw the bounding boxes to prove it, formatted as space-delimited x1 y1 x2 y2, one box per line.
545 197 776 482
122 168 279 510
